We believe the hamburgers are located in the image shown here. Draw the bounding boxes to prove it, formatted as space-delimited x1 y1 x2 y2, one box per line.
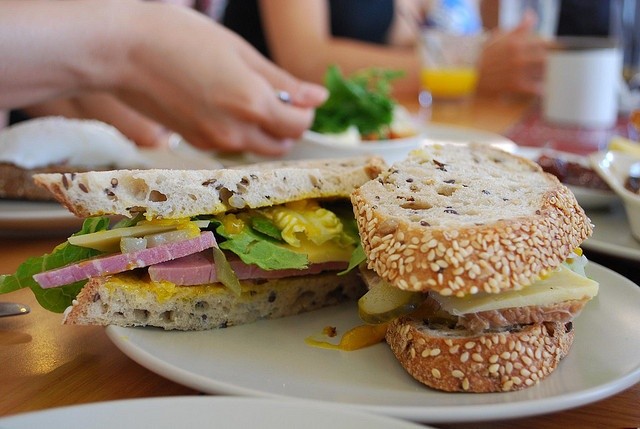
362 143 599 391
2 160 364 329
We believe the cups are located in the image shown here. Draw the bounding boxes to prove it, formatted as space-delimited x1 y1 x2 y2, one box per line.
515 35 623 129
420 26 490 99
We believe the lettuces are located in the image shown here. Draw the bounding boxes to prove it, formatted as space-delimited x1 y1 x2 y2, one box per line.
310 64 405 135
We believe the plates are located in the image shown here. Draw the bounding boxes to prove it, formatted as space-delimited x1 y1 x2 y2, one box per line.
0 394 434 429
1 196 98 244
246 117 518 164
520 141 619 216
578 204 639 262
587 142 639 241
104 260 639 420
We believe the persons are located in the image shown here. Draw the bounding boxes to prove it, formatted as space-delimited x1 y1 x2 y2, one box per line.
384 0 563 49
0 0 331 161
223 0 571 104
